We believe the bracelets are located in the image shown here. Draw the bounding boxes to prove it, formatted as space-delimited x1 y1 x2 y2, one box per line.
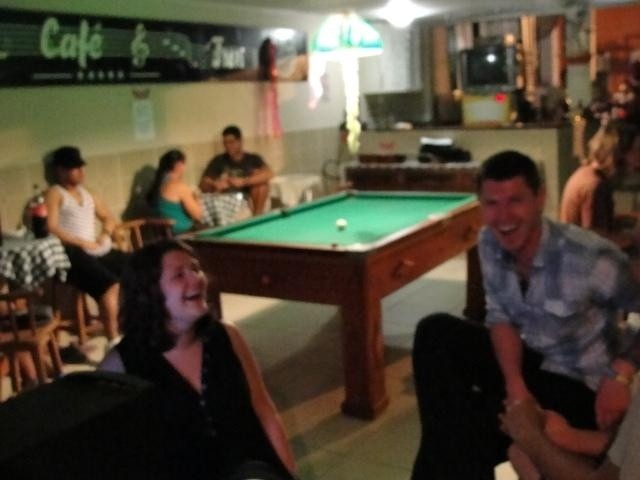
604 367 634 386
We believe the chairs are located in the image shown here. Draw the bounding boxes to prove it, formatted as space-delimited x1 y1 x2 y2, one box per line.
0 168 322 396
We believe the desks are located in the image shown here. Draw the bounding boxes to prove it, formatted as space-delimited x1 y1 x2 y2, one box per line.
172 188 486 422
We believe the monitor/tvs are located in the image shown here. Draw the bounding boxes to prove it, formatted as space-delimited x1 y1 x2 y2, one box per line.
459 45 515 95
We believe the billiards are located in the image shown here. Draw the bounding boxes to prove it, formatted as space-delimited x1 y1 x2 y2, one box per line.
336 219 347 229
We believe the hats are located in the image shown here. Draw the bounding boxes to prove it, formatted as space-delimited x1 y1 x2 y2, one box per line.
53 147 84 169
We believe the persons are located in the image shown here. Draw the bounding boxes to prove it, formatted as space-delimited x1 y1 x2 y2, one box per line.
148 148 210 236
559 124 639 250
95 237 297 480
498 369 639 480
44 146 133 354
200 126 274 216
410 148 639 480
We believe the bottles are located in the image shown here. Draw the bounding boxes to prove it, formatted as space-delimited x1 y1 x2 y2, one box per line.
30 184 50 239
